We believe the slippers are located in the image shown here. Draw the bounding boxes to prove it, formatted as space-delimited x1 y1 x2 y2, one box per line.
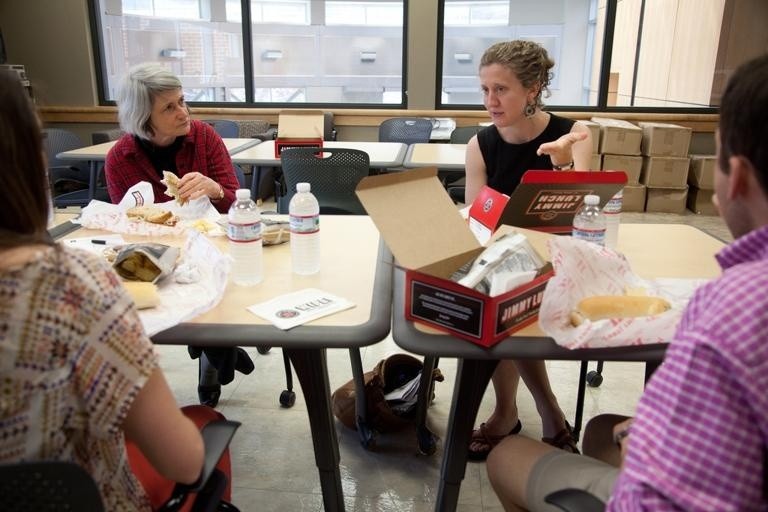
467 419 521 460
543 419 580 455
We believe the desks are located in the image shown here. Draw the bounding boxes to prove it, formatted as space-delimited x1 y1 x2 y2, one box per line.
396 216 733 508
226 134 409 206
403 138 484 173
39 209 391 508
54 131 261 207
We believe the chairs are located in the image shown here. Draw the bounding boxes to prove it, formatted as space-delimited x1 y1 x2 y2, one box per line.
376 116 434 145
271 146 375 215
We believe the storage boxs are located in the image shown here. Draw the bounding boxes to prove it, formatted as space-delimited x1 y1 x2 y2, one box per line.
647 187 689 216
687 190 720 215
690 156 718 190
591 116 644 156
603 154 644 187
570 153 602 172
642 155 692 190
619 185 647 212
574 117 601 154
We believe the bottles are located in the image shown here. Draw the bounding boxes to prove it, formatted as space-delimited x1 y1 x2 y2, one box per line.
603 168 622 248
226 187 264 286
286 181 322 277
571 195 606 246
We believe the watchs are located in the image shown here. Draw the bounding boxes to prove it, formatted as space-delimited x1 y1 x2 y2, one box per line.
550 161 575 171
209 184 225 202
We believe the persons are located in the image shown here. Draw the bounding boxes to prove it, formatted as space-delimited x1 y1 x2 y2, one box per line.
465 40 595 463
104 61 255 408
1 71 232 512
485 56 768 512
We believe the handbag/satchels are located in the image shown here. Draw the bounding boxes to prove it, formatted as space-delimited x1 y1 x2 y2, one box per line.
331 354 443 431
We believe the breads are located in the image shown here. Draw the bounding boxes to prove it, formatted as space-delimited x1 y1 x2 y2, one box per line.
568 293 675 328
119 279 159 310
127 203 177 226
160 170 190 206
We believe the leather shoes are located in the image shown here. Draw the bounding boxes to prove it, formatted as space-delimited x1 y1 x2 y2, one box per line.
198 347 220 408
